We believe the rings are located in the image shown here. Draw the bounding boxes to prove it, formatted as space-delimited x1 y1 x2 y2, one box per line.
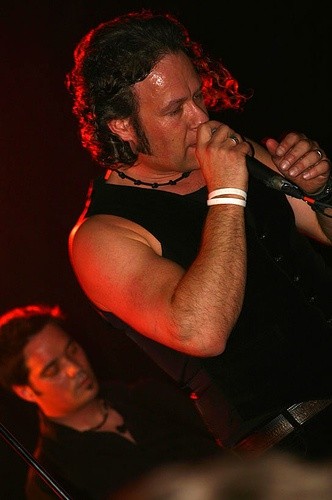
230 135 239 145
314 148 322 161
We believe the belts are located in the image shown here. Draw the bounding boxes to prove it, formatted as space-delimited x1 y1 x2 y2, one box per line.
239 396 330 460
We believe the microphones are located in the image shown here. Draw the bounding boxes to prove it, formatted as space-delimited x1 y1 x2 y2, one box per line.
212 127 321 210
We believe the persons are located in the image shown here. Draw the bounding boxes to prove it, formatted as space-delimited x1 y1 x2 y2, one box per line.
0 305 165 500
66 10 332 462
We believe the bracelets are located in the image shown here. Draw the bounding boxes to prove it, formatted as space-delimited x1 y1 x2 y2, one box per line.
307 177 332 204
207 188 247 208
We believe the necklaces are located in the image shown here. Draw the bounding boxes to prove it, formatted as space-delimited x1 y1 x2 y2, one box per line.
112 168 191 189
82 399 110 431
